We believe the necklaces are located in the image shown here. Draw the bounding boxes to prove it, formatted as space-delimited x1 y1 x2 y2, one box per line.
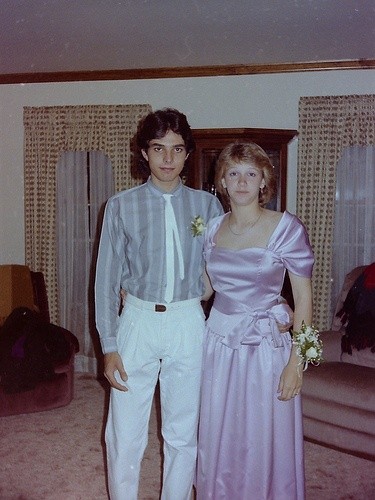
227 209 263 236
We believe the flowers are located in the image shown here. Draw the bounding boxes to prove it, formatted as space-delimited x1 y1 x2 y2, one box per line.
189 217 206 238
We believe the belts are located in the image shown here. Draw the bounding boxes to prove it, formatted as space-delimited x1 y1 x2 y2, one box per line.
125 295 200 312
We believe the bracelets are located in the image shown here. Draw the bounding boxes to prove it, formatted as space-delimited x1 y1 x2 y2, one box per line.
291 320 324 372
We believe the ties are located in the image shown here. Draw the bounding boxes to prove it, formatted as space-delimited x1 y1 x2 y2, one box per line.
161 193 184 303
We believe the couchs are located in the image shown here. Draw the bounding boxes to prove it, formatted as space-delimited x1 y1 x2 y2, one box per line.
301 264 375 460
0 264 80 416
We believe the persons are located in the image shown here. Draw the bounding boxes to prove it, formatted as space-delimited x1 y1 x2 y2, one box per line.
94 107 293 500
120 143 315 500
